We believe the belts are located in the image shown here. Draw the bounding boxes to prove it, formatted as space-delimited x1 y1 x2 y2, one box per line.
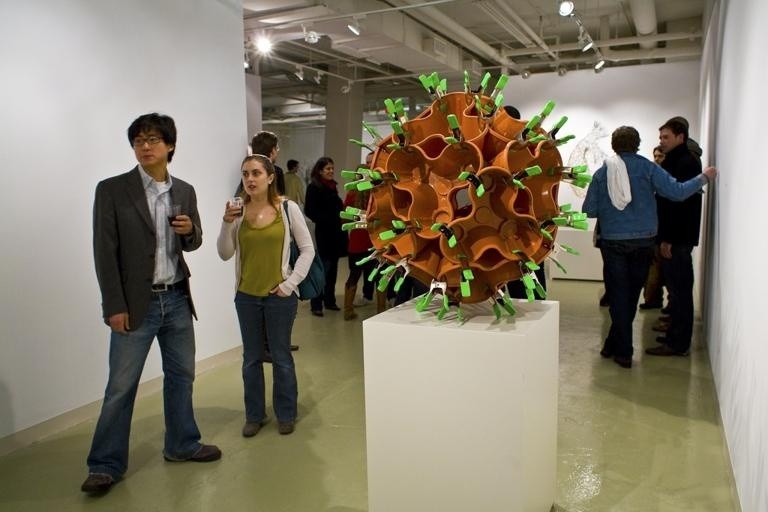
152 282 179 292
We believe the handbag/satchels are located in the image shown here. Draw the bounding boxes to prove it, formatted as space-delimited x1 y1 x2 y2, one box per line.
282 198 327 302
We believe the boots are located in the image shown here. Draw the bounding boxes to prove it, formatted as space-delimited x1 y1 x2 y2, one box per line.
344 281 359 321
375 291 385 313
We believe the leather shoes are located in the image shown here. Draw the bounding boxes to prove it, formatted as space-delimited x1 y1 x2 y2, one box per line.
312 308 325 317
324 303 341 312
645 302 691 357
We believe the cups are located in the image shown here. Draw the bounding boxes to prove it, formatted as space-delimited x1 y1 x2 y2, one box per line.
230 196 245 217
164 204 181 226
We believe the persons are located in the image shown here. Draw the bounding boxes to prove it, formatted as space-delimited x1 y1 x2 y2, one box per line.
501 106 546 300
81 113 221 498
344 151 428 320
234 132 298 362
582 117 717 368
304 157 349 317
284 160 304 204
217 155 315 437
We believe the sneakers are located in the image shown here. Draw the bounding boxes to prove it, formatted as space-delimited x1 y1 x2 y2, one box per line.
600 336 615 358
638 299 664 310
615 351 633 369
241 422 260 437
599 291 609 308
79 472 112 494
277 417 293 435
162 443 221 464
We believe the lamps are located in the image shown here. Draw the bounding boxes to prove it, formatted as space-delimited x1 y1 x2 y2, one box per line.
518 61 569 80
555 0 606 74
244 47 352 94
244 14 363 58
